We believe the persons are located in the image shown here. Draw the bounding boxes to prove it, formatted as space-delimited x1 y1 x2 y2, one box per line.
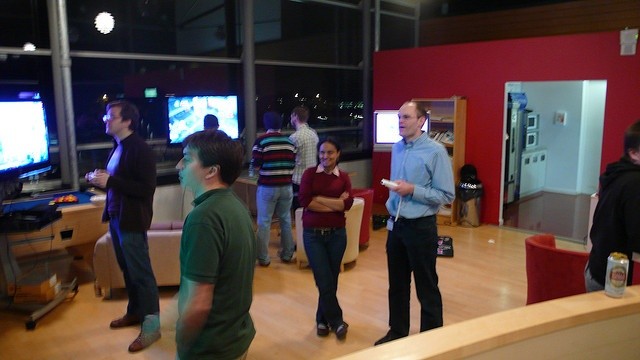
289 105 320 251
174 129 257 360
298 139 354 337
204 114 219 129
583 120 640 292
252 110 299 266
374 101 456 345
84 100 161 352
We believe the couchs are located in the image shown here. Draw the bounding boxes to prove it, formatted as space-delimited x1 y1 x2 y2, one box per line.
295 197 365 271
93 184 196 298
349 188 375 246
526 234 640 305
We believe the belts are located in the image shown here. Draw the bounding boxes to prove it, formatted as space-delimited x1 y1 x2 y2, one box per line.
304 227 345 236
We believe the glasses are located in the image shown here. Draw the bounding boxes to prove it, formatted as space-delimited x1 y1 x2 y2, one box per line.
102 114 123 123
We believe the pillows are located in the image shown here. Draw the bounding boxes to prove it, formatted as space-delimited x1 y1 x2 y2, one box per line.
149 222 172 230
172 221 184 230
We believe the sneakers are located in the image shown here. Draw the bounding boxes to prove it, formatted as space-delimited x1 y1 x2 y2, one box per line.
317 323 329 335
336 321 349 337
259 259 271 266
277 248 291 262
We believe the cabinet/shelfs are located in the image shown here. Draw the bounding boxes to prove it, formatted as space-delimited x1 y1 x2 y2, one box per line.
411 97 467 228
519 149 547 198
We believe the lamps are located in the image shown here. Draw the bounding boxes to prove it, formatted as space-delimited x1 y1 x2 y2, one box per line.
94 12 115 36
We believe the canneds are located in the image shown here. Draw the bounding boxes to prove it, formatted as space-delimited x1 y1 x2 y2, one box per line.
603 251 629 299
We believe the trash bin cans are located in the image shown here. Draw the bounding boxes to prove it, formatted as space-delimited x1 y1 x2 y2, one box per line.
457 165 483 227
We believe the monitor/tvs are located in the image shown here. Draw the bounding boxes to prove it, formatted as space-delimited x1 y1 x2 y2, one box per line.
165 94 240 147
371 110 432 152
0 99 53 197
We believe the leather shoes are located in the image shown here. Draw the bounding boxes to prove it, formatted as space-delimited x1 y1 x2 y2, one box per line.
129 332 161 352
375 330 408 346
111 315 139 327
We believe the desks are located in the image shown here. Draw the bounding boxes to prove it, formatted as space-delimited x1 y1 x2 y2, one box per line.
1 187 110 259
